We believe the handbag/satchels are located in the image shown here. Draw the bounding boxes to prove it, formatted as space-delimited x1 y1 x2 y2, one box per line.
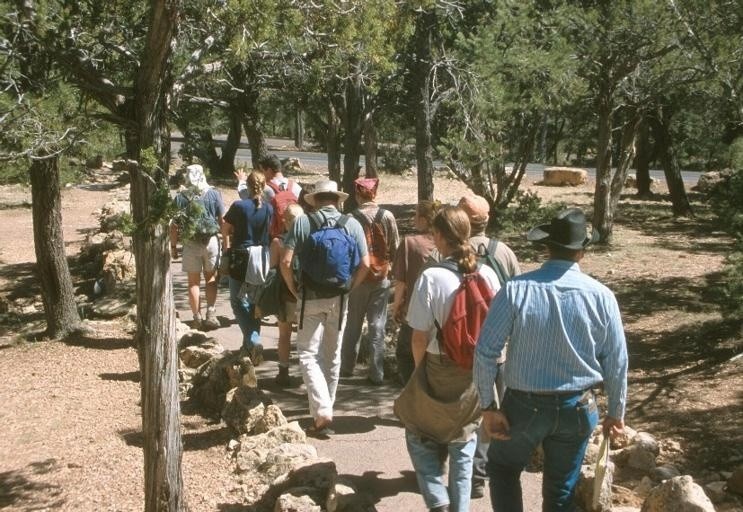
227 246 266 282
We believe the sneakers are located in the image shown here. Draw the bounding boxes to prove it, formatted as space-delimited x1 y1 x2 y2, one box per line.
192 318 204 330
204 309 221 329
250 340 264 366
471 478 485 498
306 419 335 437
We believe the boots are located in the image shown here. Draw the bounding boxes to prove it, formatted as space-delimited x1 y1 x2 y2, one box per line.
275 363 290 386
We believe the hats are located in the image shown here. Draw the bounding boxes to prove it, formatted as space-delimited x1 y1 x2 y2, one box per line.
303 178 349 207
183 163 205 186
525 206 601 253
281 203 303 226
456 194 490 226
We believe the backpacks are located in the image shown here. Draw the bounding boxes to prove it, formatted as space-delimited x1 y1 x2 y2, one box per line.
352 208 391 283
177 190 219 240
428 258 498 373
475 237 510 286
300 212 361 301
267 178 298 239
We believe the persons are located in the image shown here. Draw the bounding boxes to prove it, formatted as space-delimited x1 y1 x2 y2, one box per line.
233 153 302 236
269 203 307 387
341 177 400 388
393 207 502 512
458 195 523 499
297 184 315 212
279 179 370 438
217 171 273 368
168 164 230 332
392 200 442 381
471 207 629 512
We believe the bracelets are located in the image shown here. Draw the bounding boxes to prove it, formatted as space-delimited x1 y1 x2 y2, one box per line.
480 401 497 411
